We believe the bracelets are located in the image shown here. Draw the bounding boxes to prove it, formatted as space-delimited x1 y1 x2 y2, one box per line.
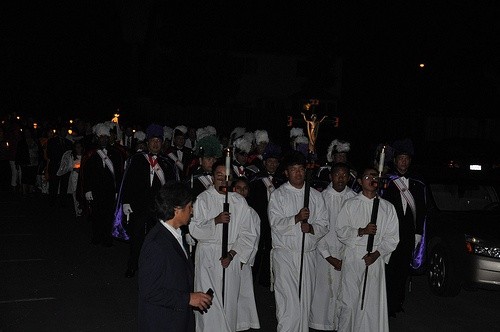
229 253 234 258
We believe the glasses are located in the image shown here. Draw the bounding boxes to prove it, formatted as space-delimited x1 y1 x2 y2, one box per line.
361 175 379 182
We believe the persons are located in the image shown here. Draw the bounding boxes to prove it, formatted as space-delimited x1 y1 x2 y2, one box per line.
335 167 400 332
0 118 419 319
267 150 330 332
188 161 261 332
309 162 357 332
137 180 213 332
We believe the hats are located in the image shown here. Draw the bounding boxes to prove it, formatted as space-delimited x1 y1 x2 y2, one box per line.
375 143 393 161
62 115 320 159
327 139 351 162
285 149 306 166
394 138 415 156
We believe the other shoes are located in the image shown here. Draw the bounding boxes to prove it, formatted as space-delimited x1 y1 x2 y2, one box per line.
93 240 114 248
388 305 396 318
121 231 130 242
395 301 405 313
124 266 136 279
